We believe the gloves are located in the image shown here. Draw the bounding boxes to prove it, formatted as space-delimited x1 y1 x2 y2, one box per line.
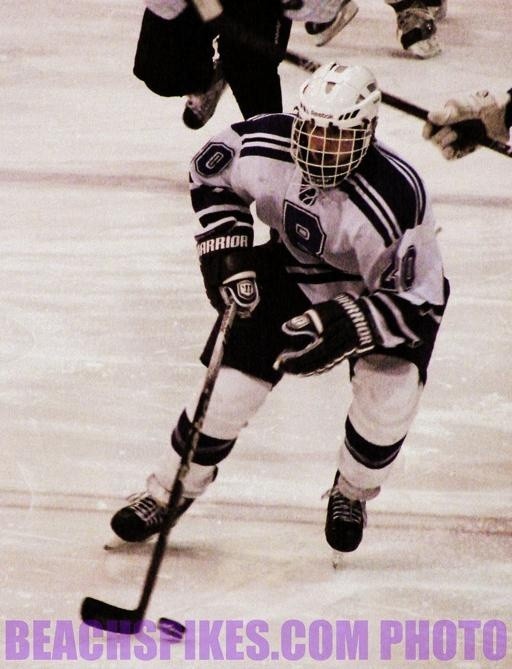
272 292 378 378
192 217 265 320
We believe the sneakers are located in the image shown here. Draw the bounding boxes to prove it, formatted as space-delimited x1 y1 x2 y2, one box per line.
182 79 229 129
394 5 438 50
304 0 351 34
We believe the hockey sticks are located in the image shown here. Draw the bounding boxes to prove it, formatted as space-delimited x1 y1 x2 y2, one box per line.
80 297 237 634
195 0 512 159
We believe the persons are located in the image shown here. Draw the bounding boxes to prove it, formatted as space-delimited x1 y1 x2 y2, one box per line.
306 0 454 58
131 0 342 130
103 52 452 555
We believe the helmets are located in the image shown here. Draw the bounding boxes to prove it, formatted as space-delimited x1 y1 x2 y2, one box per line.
288 61 381 190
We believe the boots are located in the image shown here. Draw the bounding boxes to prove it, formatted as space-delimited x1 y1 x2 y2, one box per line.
320 469 368 553
109 465 219 542
